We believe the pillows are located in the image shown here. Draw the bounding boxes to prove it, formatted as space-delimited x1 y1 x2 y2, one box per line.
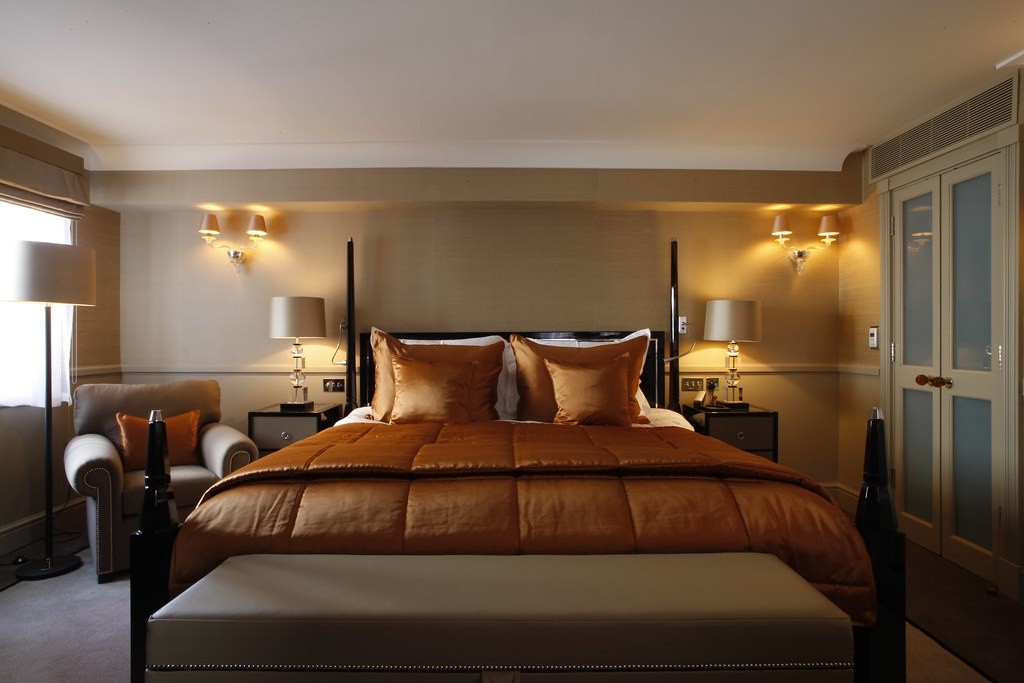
509 333 651 425
503 327 652 418
544 357 633 428
368 327 520 418
117 409 201 472
390 355 479 426
364 331 506 422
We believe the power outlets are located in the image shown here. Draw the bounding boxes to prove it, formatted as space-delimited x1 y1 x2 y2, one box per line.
706 378 718 390
679 316 687 334
340 317 348 335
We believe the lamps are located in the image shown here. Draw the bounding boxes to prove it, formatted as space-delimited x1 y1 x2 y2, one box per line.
771 215 840 278
1 241 98 581
198 214 268 279
663 322 696 364
703 300 762 411
332 322 348 366
268 297 326 412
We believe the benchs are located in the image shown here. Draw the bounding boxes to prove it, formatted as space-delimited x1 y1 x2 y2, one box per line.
143 553 855 683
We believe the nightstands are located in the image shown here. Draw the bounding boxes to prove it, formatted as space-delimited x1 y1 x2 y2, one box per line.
683 402 779 464
248 404 342 458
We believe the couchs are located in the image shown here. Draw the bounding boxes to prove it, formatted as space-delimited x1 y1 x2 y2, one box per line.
64 380 259 584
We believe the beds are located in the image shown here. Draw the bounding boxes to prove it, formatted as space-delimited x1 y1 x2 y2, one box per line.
129 333 907 683
